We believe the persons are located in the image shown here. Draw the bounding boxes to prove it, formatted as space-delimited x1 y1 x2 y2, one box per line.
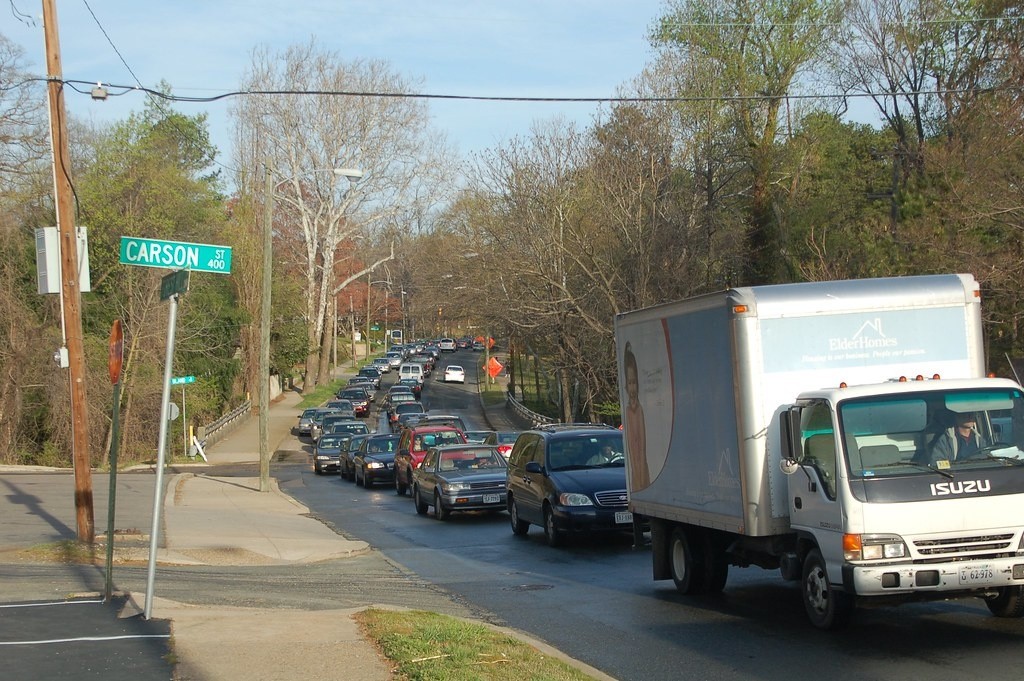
472 457 498 470
371 444 382 453
928 413 986 466
622 339 650 492
412 435 427 451
585 438 624 468
437 437 456 446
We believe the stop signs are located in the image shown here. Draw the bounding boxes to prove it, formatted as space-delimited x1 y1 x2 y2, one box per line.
109 320 123 385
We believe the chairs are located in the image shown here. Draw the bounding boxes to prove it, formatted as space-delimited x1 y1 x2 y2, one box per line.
806 433 902 503
549 450 569 467
922 408 957 464
440 459 458 471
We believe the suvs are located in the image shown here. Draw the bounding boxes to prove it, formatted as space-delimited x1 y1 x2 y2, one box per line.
393 423 468 497
505 422 650 545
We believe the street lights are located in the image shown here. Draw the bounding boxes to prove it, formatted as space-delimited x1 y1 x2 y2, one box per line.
258 168 364 494
365 280 393 359
385 289 406 353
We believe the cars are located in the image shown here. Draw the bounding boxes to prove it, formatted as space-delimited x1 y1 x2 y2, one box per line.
296 338 486 441
339 435 374 482
482 431 524 463
462 430 493 443
415 415 466 432
412 443 507 520
313 432 353 473
354 433 401 488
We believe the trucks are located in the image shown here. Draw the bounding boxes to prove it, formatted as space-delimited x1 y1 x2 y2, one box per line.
611 272 1024 628
